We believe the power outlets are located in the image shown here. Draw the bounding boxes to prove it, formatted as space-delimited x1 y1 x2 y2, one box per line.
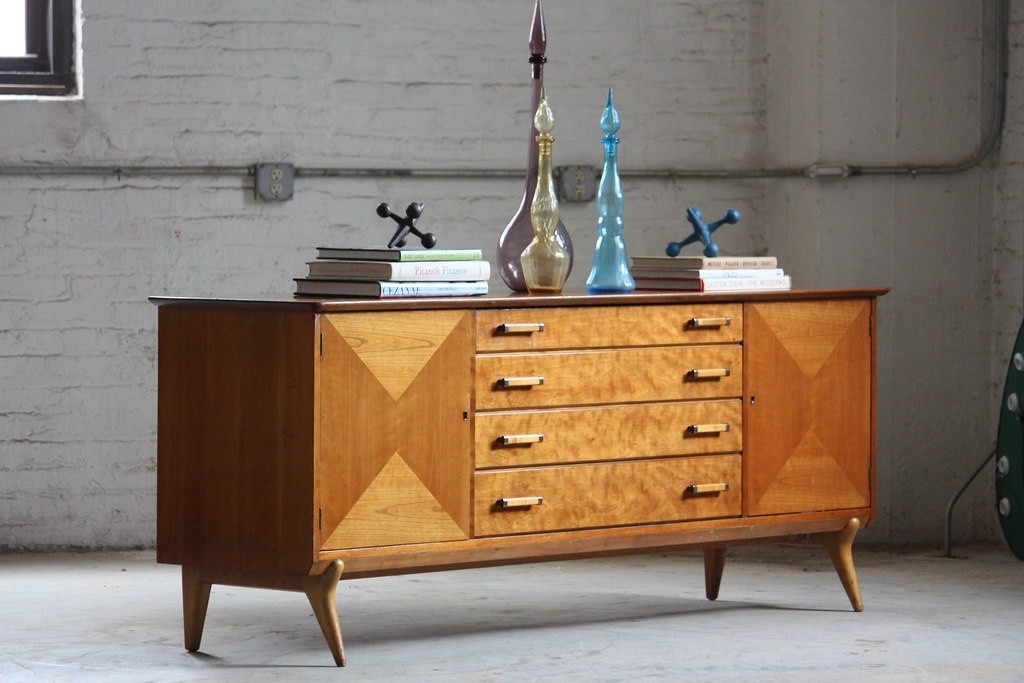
254 161 293 201
555 164 595 202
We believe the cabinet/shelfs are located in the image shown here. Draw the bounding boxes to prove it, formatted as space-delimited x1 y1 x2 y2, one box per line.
146 287 891 668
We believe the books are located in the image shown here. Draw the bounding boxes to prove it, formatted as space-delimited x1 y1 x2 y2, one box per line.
293 246 490 298
628 256 791 292
